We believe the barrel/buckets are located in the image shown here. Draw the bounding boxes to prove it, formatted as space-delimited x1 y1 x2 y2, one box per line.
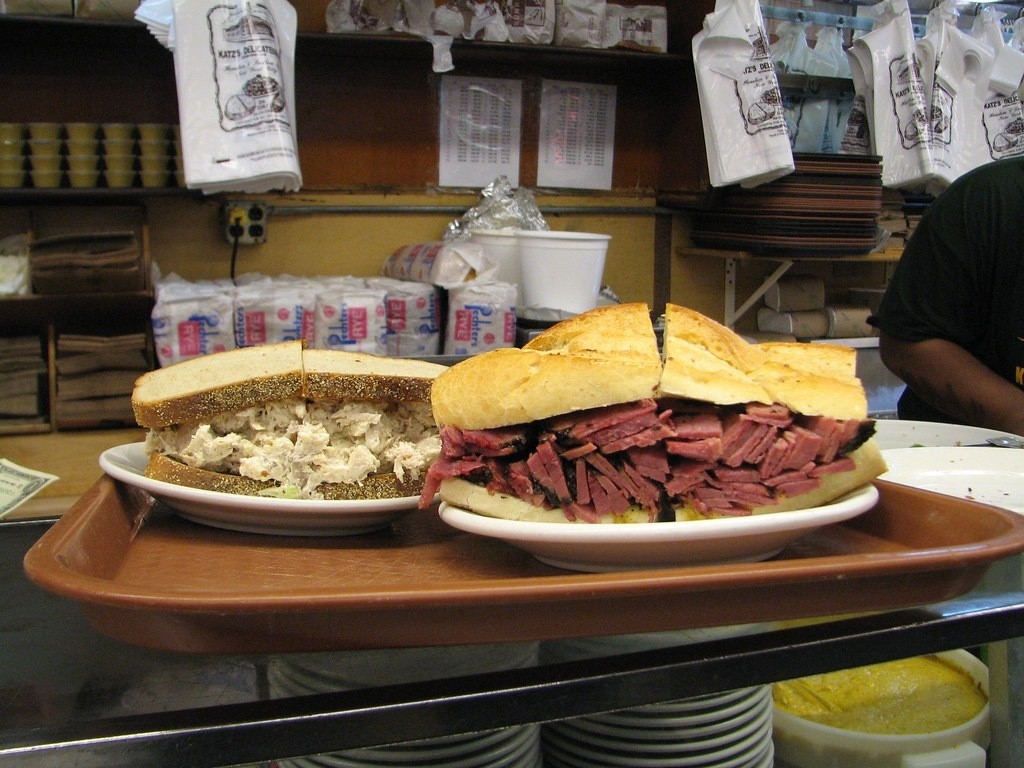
770 648 989 768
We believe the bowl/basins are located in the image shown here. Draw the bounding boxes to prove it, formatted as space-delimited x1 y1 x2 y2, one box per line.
0 120 185 188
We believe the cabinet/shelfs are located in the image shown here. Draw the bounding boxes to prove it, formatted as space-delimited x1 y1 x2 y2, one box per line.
0 0 904 348
0 517 1024 768
0 224 154 436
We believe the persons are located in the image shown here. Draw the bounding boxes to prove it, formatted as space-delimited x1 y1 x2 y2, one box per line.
866 156 1024 436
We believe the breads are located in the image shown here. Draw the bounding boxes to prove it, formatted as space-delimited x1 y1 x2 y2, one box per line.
132 303 887 522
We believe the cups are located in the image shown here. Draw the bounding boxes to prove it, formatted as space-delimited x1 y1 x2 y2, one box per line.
516 229 612 315
469 228 522 284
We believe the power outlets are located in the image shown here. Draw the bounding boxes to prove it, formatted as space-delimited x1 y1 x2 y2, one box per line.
224 199 269 245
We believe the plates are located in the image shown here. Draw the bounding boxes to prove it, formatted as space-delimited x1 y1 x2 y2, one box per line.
439 482 879 572
695 152 884 254
99 441 438 537
120 628 775 768
877 445 1024 517
873 419 1024 451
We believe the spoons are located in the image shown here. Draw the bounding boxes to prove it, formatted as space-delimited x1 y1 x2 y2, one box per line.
986 437 1024 449
